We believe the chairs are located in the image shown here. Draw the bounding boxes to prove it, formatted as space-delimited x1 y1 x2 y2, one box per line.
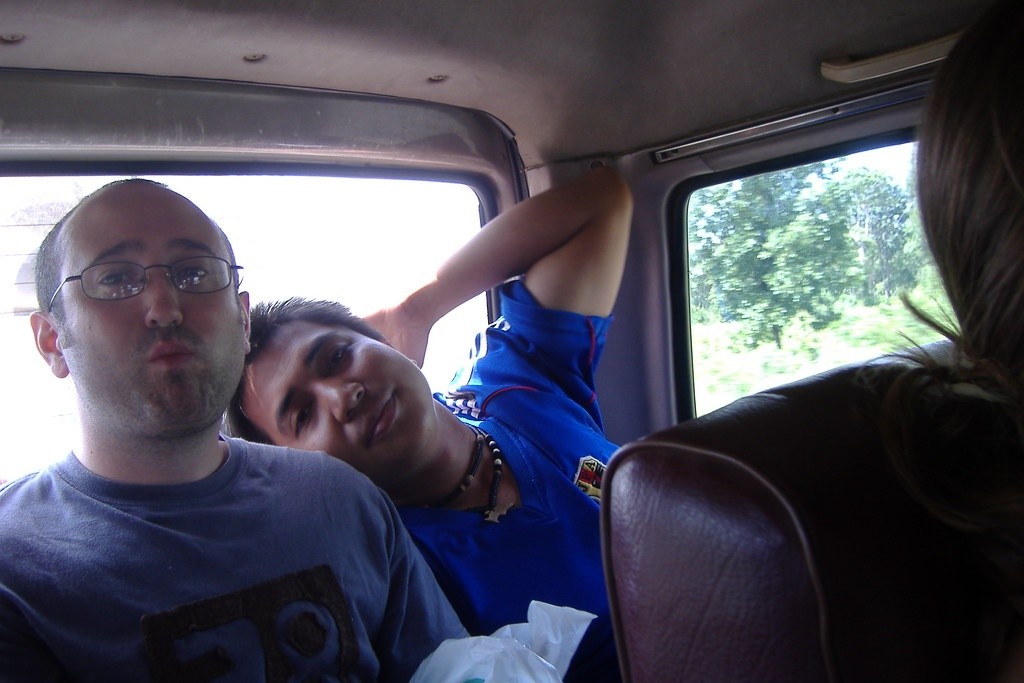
599 343 955 683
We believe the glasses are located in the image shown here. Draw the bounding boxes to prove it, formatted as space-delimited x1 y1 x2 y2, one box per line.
47 256 244 310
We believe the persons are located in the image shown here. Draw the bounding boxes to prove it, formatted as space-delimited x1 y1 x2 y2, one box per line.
880 0 1024 683
0 178 471 683
224 164 635 682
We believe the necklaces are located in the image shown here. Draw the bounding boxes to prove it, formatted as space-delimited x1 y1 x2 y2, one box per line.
435 424 503 513
